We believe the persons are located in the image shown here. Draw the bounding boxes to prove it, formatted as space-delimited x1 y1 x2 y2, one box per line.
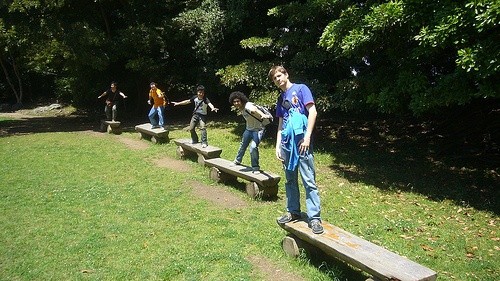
98 82 127 122
171 85 217 147
229 92 270 175
268 66 323 234
148 82 165 130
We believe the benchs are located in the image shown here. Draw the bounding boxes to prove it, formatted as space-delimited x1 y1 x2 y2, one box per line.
134 123 168 144
204 157 281 201
173 139 223 165
282 218 437 281
100 118 120 133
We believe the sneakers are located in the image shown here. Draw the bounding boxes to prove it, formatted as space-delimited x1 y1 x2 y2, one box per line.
277 213 301 223
308 219 323 233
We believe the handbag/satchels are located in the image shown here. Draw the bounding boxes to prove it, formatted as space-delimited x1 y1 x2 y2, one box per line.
244 103 274 123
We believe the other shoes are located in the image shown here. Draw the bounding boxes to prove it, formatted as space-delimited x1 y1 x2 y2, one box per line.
229 161 237 166
189 141 195 143
160 127 164 130
253 170 259 174
202 144 207 147
